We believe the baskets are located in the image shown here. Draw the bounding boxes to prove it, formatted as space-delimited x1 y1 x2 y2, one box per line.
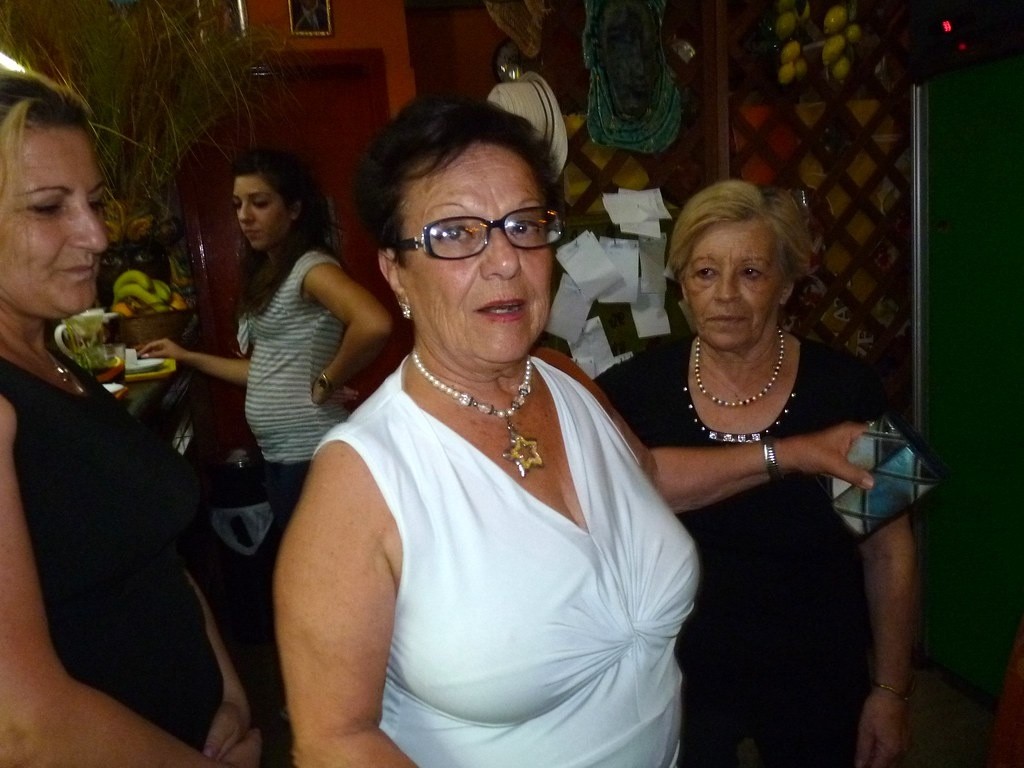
106 306 192 349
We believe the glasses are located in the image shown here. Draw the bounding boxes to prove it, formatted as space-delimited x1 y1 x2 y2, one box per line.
394 204 565 261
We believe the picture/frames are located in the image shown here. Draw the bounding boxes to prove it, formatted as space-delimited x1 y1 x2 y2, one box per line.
288 0 333 38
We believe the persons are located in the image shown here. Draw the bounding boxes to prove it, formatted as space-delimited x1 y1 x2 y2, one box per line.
274 90 875 768
0 72 263 768
591 179 920 768
135 145 394 541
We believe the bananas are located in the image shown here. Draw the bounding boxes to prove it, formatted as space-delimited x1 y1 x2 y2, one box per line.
112 269 172 313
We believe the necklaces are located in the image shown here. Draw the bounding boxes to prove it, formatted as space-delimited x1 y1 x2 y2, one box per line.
694 326 785 407
411 347 546 478
32 349 71 382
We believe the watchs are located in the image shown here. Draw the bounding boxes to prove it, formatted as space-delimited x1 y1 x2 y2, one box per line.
318 368 337 393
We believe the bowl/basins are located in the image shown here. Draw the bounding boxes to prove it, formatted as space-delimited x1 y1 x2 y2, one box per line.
113 307 193 350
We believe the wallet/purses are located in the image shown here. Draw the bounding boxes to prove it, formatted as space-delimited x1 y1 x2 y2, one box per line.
815 412 948 538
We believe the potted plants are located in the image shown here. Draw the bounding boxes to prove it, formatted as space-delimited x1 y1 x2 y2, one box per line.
0 0 314 306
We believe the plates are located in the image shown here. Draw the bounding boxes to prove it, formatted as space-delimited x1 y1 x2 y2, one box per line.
125 358 166 375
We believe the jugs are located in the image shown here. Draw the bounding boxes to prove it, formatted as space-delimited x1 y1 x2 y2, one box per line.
54 309 123 382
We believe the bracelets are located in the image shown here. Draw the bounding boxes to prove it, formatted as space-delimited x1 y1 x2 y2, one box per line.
761 435 780 477
872 670 918 701
